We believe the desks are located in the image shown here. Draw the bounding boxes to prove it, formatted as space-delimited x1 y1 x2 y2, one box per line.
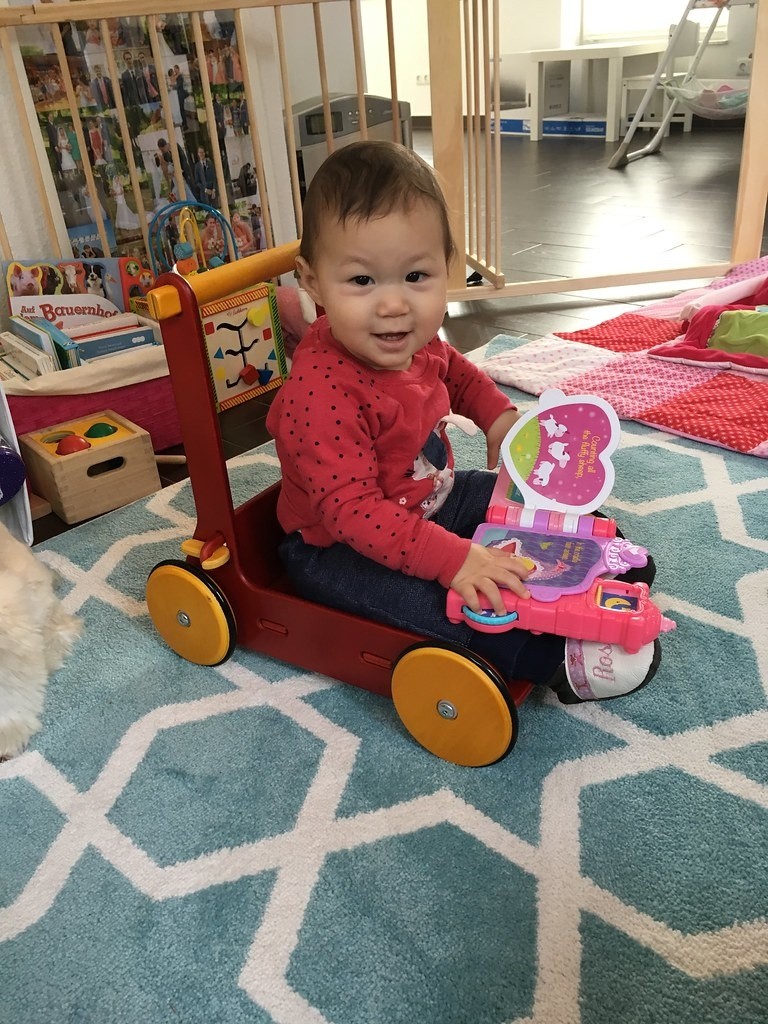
525 38 668 143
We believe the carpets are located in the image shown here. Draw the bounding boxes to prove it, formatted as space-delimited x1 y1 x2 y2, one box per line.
0 334 767 1024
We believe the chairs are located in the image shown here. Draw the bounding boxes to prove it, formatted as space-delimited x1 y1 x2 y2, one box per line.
146 241 626 767
620 20 700 138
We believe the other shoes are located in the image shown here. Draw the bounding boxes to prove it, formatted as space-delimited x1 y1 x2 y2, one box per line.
598 555 656 587
545 638 661 705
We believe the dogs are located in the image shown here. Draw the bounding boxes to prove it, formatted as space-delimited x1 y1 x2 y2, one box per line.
80 261 107 296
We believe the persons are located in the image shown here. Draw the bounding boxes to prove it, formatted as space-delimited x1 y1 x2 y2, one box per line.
58 19 116 113
165 217 181 264
150 138 216 211
193 9 250 137
46 113 84 173
266 140 662 705
36 70 68 107
81 118 113 167
194 204 267 268
237 162 260 198
155 12 189 131
81 244 105 259
106 16 161 108
68 170 156 230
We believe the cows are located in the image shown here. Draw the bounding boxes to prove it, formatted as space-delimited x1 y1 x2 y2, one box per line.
60 261 83 295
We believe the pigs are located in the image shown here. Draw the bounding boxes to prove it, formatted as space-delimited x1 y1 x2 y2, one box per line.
11 265 61 297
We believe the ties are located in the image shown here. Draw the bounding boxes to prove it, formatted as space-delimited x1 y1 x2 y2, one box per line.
168 150 171 154
203 162 207 172
99 80 109 105
131 70 135 80
